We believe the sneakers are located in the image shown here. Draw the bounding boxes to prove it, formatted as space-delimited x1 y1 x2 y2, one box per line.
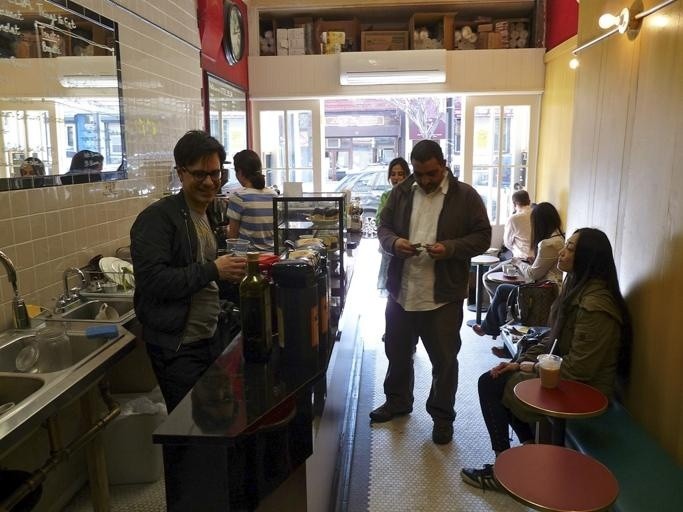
460 464 502 493
369 401 412 422
432 417 454 444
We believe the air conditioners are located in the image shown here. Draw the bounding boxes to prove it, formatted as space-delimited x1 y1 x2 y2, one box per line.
55 54 116 88
337 49 447 87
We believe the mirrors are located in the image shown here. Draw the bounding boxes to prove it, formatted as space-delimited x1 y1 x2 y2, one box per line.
206 72 249 161
0 0 128 191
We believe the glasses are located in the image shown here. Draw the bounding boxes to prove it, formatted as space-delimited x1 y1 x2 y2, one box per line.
180 165 224 181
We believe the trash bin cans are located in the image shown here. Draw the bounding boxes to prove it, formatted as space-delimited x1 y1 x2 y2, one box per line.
88 392 166 485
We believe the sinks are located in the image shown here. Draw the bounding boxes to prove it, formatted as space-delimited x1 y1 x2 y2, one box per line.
0 334 124 374
0 376 45 419
63 300 135 320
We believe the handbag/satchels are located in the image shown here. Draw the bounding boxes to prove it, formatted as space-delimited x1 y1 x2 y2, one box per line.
505 280 559 326
510 326 552 362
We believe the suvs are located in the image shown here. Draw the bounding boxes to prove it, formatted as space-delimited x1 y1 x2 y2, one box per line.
329 163 497 241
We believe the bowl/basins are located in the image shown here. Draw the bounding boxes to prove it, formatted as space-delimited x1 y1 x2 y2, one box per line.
305 216 339 225
24 303 52 329
116 246 132 264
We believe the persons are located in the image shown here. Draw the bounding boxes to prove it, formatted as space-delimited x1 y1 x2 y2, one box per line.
63 150 103 175
369 140 492 445
460 228 633 494
129 130 247 415
471 202 565 341
481 190 534 301
19 156 45 177
225 150 282 252
375 157 411 342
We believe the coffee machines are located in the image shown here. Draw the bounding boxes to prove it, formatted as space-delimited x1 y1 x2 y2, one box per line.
206 169 230 234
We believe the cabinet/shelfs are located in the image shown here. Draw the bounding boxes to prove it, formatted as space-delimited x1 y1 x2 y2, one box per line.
272 192 348 314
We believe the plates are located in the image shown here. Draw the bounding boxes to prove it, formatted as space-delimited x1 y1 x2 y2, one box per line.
503 272 518 278
298 235 313 239
98 256 135 287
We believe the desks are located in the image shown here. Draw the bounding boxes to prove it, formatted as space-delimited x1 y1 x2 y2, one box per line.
465 254 500 328
493 444 621 510
486 270 528 317
511 379 609 445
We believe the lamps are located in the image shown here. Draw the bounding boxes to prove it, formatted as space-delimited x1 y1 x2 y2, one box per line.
598 6 631 36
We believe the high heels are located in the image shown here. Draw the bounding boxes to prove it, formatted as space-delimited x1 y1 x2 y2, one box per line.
492 347 511 358
472 325 497 340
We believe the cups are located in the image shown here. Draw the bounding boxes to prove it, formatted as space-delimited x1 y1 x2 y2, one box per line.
93 302 118 321
501 263 513 272
537 354 562 389
225 239 249 257
0 401 14 414
101 283 117 293
507 268 519 276
35 323 73 374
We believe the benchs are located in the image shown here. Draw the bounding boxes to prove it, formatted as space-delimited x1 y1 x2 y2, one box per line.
497 325 683 511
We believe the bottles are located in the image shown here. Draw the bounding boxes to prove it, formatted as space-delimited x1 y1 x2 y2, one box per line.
243 357 270 425
238 250 273 366
13 294 29 329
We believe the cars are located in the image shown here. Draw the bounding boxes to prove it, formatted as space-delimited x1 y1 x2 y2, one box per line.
328 159 347 180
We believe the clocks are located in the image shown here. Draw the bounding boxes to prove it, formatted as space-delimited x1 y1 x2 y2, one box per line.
222 5 246 65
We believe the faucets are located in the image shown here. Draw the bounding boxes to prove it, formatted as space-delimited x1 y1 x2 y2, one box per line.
0 251 19 295
63 266 85 300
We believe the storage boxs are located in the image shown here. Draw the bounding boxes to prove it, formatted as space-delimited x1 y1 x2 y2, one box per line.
409 10 458 50
474 32 501 49
361 29 409 50
314 17 359 56
